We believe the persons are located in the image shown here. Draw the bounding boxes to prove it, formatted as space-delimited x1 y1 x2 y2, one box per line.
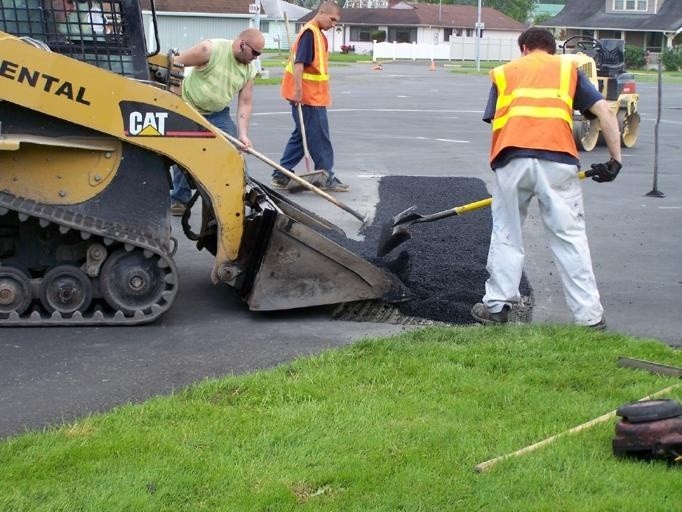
268 0 349 195
162 26 266 221
470 27 624 332
2 0 89 47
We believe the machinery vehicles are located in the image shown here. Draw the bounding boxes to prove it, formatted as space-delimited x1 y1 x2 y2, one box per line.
558 34 641 153
0 0 414 328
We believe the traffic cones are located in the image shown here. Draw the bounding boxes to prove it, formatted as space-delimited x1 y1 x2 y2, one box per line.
369 64 383 70
430 61 436 72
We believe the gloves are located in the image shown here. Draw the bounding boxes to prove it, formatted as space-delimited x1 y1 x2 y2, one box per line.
592 159 623 182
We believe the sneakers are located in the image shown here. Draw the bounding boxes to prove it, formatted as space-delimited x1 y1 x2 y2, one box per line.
172 201 186 218
317 171 352 193
468 301 508 326
590 313 608 330
271 175 292 188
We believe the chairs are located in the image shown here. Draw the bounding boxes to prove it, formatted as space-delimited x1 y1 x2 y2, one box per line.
595 38 625 76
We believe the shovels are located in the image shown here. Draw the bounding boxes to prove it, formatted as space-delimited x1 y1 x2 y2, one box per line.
376 164 610 258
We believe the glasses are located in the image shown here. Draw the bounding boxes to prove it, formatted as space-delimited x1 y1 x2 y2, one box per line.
246 43 260 57
